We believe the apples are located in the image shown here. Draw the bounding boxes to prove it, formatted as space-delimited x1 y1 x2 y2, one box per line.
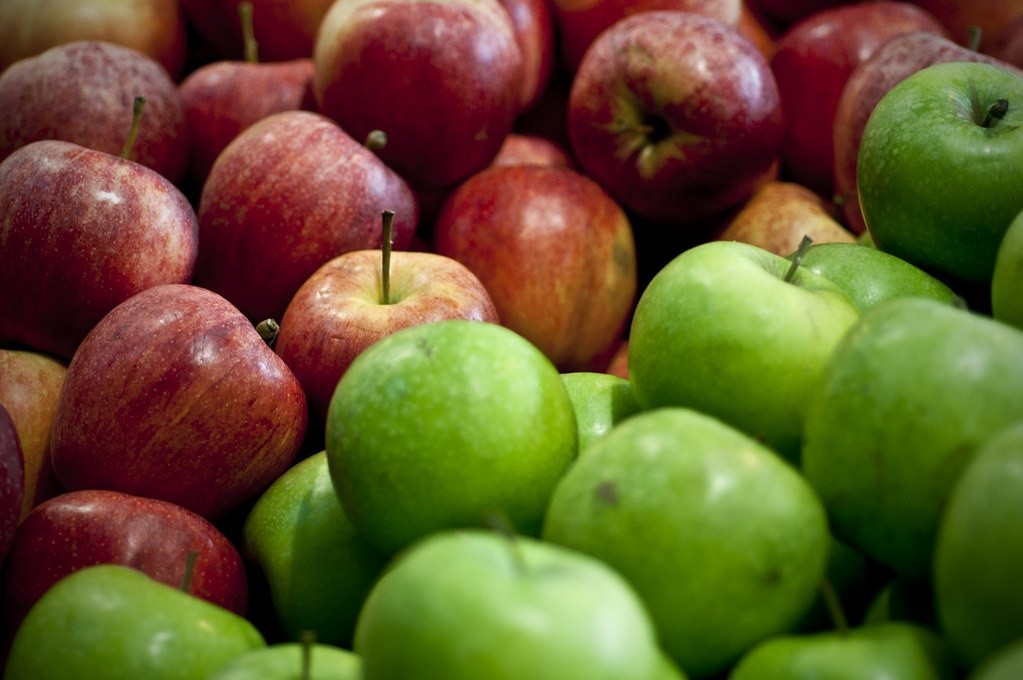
0 0 1023 680
